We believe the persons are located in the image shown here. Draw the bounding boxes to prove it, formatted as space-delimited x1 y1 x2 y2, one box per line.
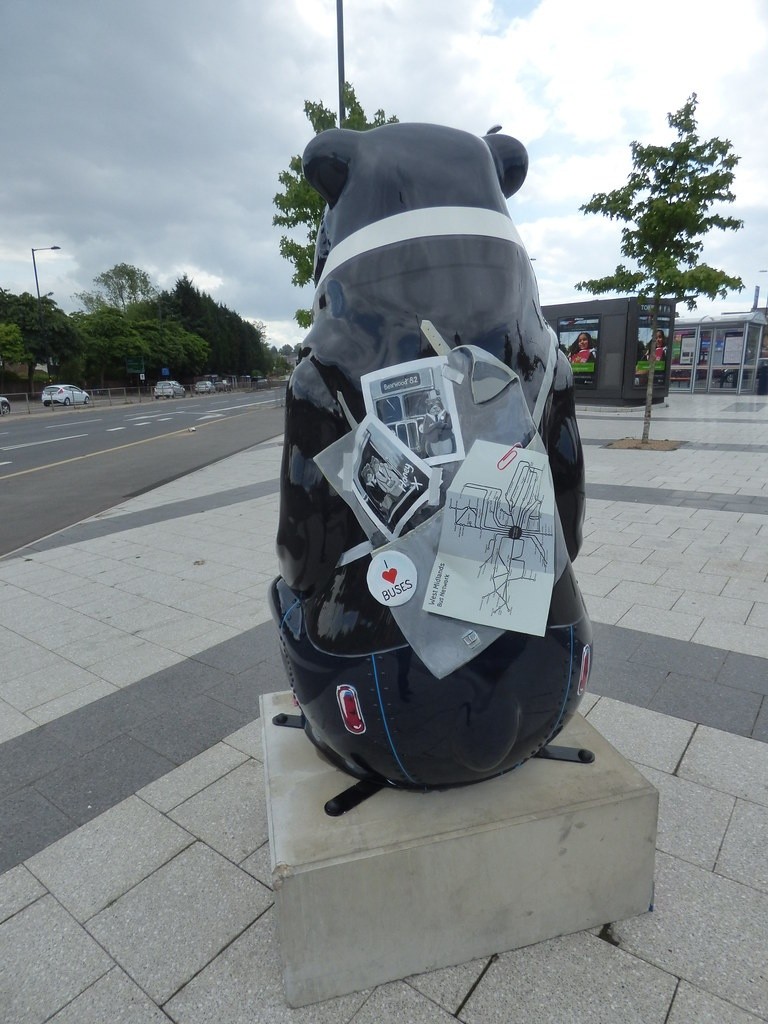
567 332 597 363
642 330 669 361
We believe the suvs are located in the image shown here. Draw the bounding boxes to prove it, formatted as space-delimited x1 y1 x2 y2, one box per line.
153 381 185 400
41 384 91 406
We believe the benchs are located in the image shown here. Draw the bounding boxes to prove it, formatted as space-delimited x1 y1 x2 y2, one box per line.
671 377 691 390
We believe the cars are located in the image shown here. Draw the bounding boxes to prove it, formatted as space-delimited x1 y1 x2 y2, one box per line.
256 379 272 390
715 357 768 382
194 380 216 395
0 397 13 417
213 378 233 392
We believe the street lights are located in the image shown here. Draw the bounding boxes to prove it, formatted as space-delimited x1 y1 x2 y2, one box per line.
32 246 62 385
690 315 715 393
